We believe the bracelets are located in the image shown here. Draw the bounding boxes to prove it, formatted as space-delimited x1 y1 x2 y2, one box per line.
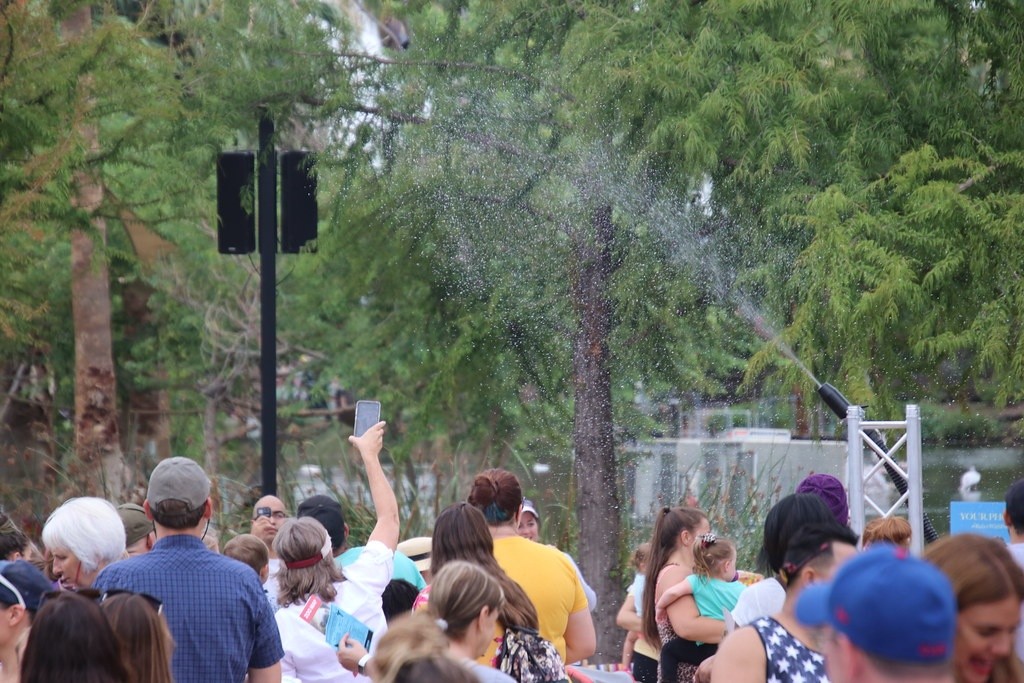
358 654 371 675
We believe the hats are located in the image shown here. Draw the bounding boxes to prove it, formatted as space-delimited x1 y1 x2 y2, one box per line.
117 503 154 547
298 495 343 532
147 456 210 516
0 559 54 611
795 474 849 526
795 542 958 666
397 537 433 571
521 498 539 518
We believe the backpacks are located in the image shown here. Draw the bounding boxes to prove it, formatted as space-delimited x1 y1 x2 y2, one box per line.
497 624 569 683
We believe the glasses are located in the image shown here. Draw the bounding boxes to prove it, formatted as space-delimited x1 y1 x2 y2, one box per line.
780 542 827 588
37 588 100 612
101 589 163 614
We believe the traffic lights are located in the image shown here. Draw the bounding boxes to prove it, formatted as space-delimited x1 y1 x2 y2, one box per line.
278 151 319 253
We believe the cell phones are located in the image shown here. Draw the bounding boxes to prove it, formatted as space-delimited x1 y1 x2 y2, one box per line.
257 507 271 519
354 400 381 438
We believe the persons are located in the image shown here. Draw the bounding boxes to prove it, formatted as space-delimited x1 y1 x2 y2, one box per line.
518 499 597 612
20 590 174 683
615 542 660 683
862 515 913 558
41 496 130 589
1 515 78 683
731 475 847 630
792 544 957 683
396 503 541 633
641 506 749 683
1006 479 1024 668
474 619 570 683
91 455 285 683
467 468 596 665
923 533 1023 683
274 420 433 683
711 521 860 683
335 560 518 683
